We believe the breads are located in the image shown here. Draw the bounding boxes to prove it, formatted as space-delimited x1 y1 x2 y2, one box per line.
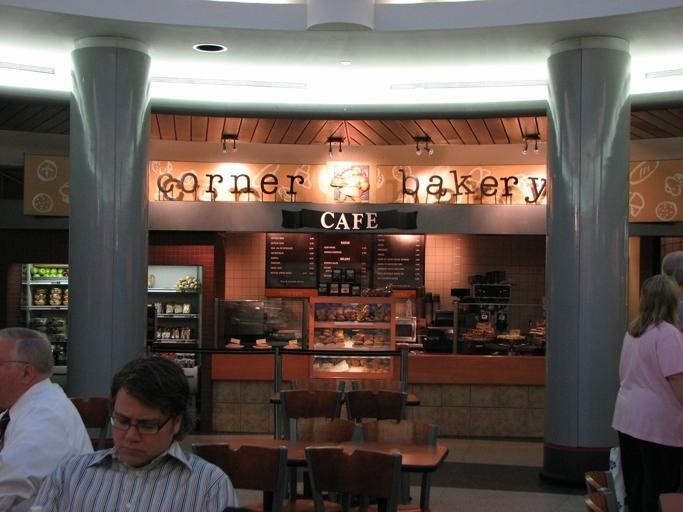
313 308 390 373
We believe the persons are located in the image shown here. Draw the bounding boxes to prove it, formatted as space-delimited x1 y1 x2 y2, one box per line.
27 358 240 512
0 326 96 512
660 250 682 287
610 275 682 512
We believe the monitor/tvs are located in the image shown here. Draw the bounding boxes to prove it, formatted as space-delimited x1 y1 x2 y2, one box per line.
435 310 476 328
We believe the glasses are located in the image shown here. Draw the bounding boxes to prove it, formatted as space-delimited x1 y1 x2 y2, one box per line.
107 406 178 437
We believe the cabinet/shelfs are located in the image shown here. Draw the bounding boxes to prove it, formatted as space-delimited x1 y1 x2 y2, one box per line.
19 263 203 377
308 297 397 378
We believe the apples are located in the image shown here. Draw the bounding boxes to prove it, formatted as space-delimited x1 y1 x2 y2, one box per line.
31 267 64 278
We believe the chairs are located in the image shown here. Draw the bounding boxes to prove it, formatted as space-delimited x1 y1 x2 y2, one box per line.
584 469 623 512
192 379 439 512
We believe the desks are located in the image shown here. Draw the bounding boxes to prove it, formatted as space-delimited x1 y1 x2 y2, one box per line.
181 434 450 512
